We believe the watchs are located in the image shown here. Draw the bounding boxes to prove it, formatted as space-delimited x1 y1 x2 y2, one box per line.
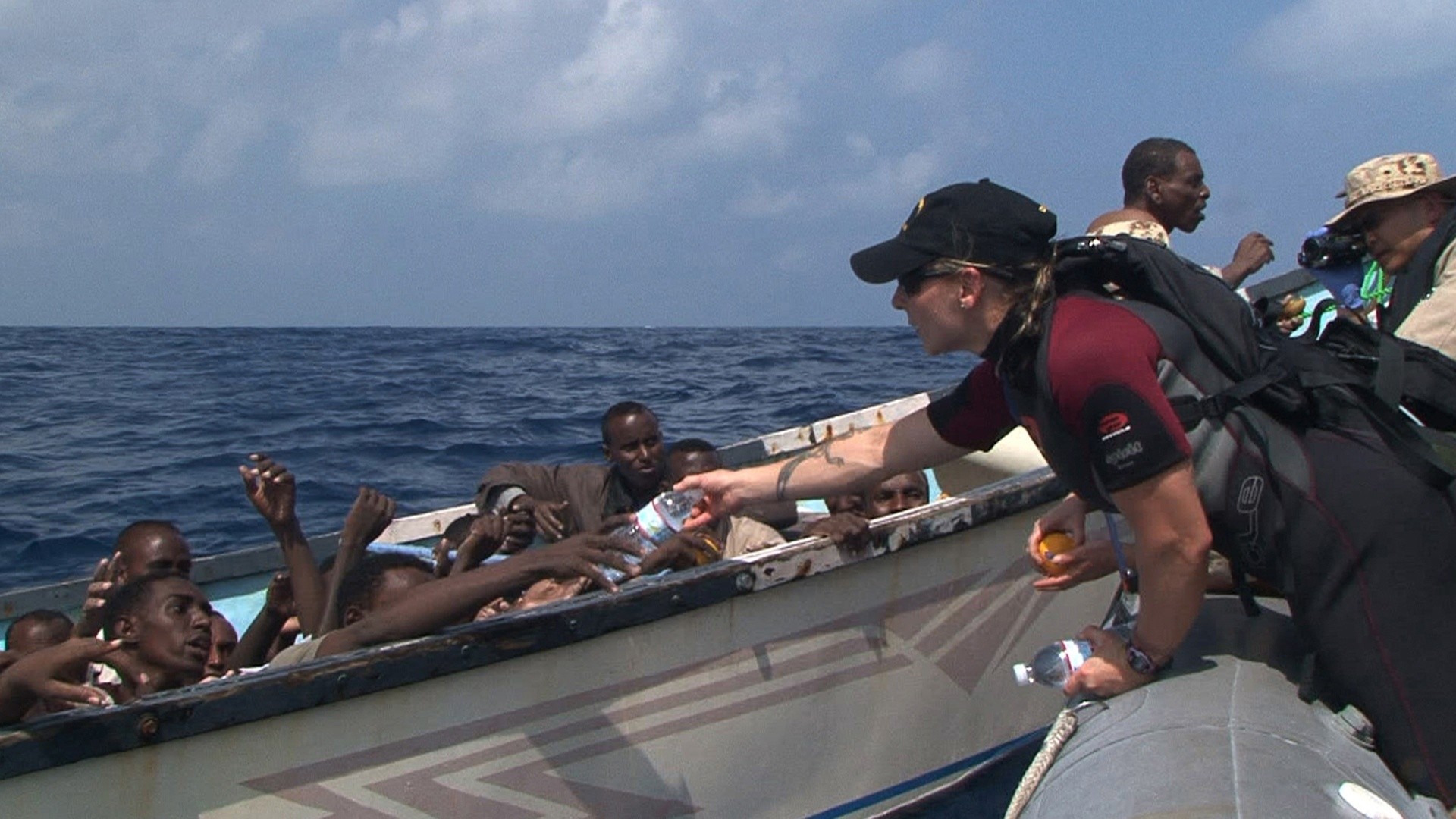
1123 628 1173 675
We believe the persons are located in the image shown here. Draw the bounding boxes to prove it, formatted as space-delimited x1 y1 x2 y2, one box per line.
1026 490 1284 595
1299 152 1456 361
1084 138 1306 338
674 179 1456 811
0 399 946 725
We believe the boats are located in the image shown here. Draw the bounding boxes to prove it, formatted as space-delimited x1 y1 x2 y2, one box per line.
0 246 1398 819
1004 593 1455 819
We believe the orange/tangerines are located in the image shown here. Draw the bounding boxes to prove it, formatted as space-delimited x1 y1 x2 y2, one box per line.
695 538 722 567
1038 532 1076 577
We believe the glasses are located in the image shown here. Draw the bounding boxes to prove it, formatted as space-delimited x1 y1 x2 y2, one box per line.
898 270 954 299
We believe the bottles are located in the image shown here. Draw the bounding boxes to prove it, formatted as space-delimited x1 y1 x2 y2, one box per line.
1014 622 1140 689
596 488 705 584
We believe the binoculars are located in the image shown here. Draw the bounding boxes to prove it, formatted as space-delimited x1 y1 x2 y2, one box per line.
1296 231 1369 268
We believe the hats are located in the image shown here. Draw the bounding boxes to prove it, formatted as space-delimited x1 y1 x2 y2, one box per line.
849 178 1058 285
1323 153 1456 231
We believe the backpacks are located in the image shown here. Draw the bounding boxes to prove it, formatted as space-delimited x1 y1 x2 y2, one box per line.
1320 316 1455 432
1032 233 1317 619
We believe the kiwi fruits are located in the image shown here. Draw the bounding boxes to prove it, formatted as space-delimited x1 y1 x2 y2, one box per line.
1282 299 1306 319
